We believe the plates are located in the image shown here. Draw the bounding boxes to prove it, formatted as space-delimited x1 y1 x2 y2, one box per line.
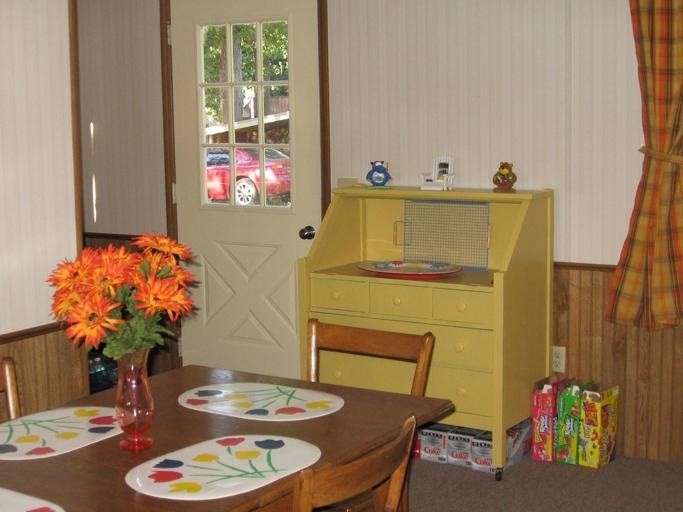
356 261 464 279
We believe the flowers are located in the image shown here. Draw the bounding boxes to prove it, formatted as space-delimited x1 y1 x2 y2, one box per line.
47 231 202 359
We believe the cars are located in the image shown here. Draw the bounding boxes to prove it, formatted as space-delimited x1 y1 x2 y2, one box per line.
206 147 291 206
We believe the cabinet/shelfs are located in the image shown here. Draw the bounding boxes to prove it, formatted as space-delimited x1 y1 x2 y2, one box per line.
297 186 556 483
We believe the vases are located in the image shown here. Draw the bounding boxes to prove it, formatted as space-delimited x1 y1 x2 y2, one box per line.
114 349 154 452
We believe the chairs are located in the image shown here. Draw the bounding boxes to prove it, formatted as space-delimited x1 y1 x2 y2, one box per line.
291 415 417 511
306 317 436 511
0 357 22 419
